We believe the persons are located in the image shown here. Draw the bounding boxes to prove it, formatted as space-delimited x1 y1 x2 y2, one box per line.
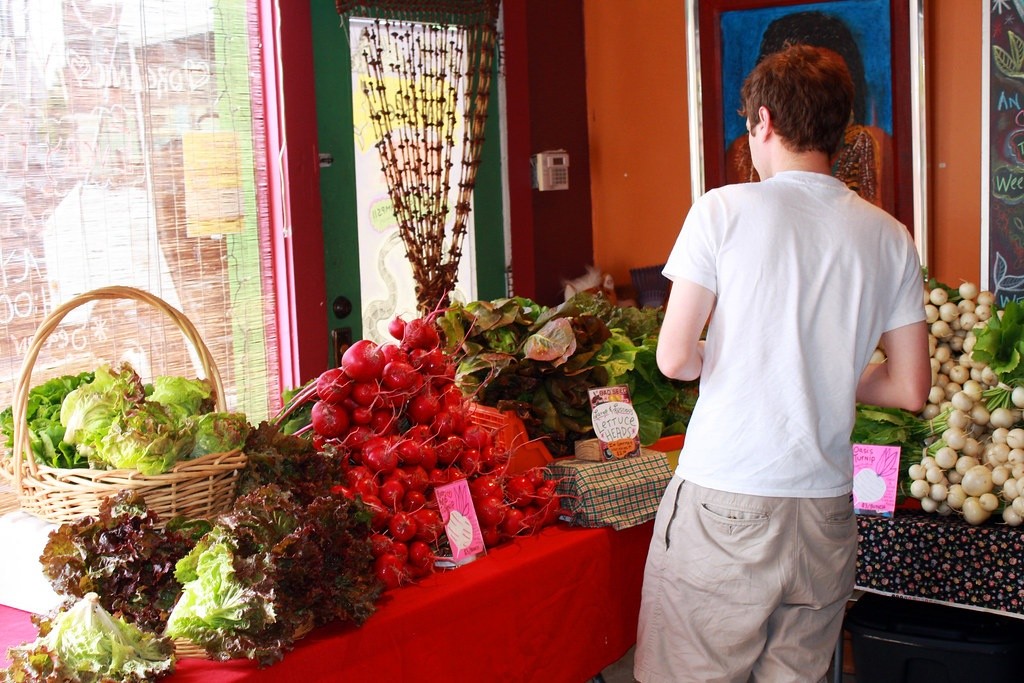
632 43 932 683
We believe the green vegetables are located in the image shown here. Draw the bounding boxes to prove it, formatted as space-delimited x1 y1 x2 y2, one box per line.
428 293 702 455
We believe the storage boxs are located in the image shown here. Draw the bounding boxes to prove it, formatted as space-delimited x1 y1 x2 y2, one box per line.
840 591 1024 683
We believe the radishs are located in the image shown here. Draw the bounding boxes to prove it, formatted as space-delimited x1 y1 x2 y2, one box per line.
867 282 1024 528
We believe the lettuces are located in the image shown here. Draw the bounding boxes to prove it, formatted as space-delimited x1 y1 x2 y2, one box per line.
0 359 388 683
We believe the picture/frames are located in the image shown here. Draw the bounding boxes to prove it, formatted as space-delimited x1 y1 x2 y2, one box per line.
684 0 931 280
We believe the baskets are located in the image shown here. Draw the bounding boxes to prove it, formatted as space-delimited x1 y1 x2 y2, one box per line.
0 285 247 529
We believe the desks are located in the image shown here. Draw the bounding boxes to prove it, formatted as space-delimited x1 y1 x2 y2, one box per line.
831 512 1024 683
1 512 664 683
